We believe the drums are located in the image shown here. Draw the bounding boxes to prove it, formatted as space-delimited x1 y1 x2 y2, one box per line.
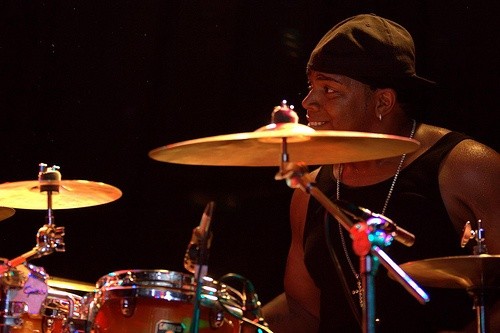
2 269 99 333
89 269 244 333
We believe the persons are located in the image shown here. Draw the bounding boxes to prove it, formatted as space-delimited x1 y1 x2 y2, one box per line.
260 14 500 333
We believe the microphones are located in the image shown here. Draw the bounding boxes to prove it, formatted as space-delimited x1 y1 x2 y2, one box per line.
334 199 416 247
183 201 215 274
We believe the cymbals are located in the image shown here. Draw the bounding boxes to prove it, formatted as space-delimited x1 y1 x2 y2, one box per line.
0 179 122 210
148 121 421 168
387 253 500 289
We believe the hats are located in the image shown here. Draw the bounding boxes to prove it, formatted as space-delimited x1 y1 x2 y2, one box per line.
307 11 438 89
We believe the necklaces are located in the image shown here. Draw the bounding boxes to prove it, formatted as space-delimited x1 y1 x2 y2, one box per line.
336 120 416 308
